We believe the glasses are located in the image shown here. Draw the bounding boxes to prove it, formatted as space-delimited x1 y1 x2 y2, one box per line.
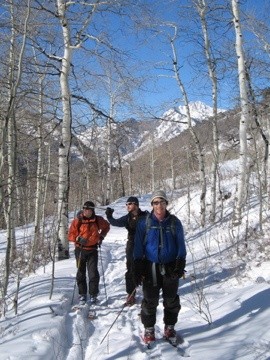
153 201 166 205
126 202 134 206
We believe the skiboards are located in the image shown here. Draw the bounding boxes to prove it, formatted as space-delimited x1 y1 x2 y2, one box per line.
122 294 140 313
138 325 191 360
77 304 96 319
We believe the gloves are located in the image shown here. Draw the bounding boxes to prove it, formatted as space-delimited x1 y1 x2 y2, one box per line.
168 261 186 280
78 237 88 244
105 207 114 218
98 236 104 245
131 259 144 286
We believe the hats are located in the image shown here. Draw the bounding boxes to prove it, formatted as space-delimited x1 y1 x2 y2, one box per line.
127 197 139 204
83 201 94 209
150 191 169 204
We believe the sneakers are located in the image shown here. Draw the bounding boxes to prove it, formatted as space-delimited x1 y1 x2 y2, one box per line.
164 325 177 342
79 296 87 306
90 298 95 307
144 327 155 344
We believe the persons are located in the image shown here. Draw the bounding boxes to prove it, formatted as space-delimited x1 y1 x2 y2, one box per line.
106 197 149 307
66 201 111 304
129 189 187 345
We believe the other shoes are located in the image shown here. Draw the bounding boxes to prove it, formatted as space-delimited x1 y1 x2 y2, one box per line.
127 295 134 304
